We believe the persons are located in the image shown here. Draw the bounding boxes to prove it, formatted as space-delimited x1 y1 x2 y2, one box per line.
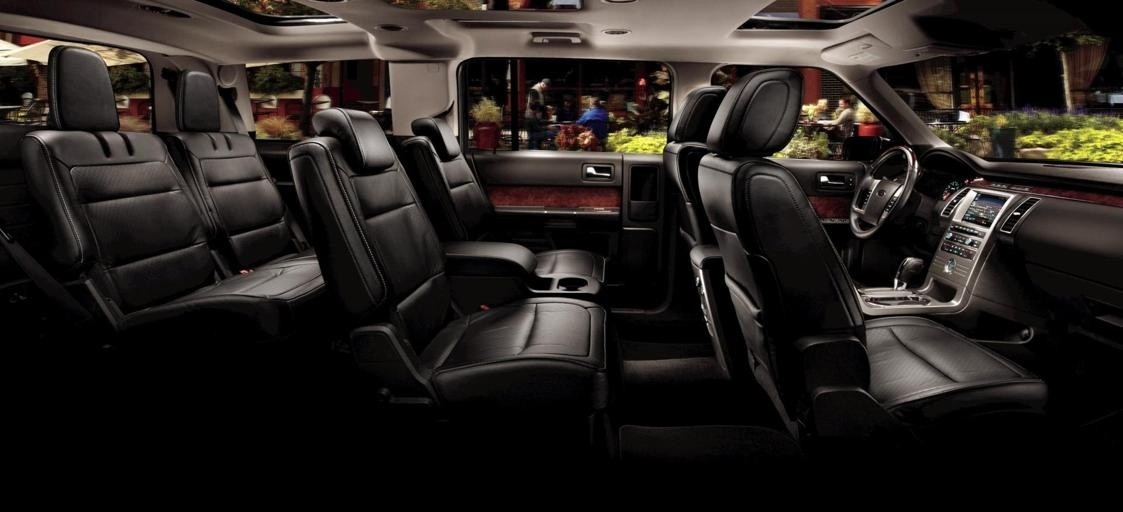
810 97 855 142
556 95 576 121
544 104 557 122
524 78 551 149
575 97 609 151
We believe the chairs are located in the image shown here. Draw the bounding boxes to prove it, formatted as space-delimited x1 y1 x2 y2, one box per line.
19 43 327 361
706 63 1050 509
287 105 618 480
159 69 320 266
402 117 611 312
662 85 750 379
827 121 854 160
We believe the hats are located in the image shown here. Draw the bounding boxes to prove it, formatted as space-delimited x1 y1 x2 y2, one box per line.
542 77 552 87
